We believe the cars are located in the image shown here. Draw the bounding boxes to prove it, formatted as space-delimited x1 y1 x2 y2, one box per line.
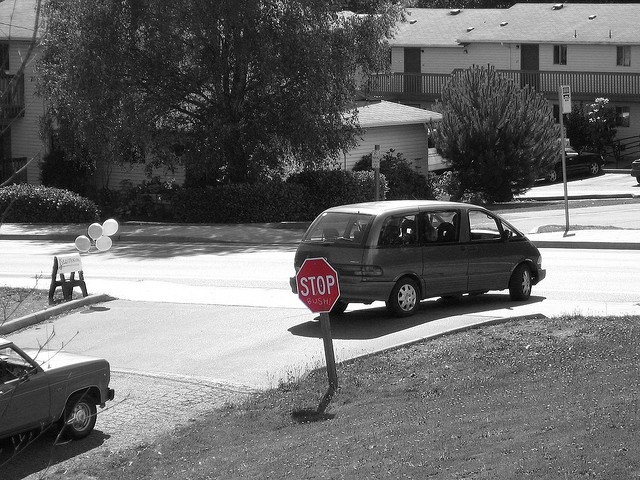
0 337 115 442
427 129 453 171
631 159 640 183
535 147 606 184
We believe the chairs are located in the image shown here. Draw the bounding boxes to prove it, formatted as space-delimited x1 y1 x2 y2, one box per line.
402 220 415 242
438 222 455 242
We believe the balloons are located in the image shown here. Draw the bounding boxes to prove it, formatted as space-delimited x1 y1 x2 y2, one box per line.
102 218 119 236
87 223 103 240
96 236 112 252
74 235 91 253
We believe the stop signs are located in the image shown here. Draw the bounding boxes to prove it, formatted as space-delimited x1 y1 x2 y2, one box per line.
296 257 341 313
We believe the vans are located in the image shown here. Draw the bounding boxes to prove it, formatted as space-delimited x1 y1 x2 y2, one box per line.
290 199 546 318
